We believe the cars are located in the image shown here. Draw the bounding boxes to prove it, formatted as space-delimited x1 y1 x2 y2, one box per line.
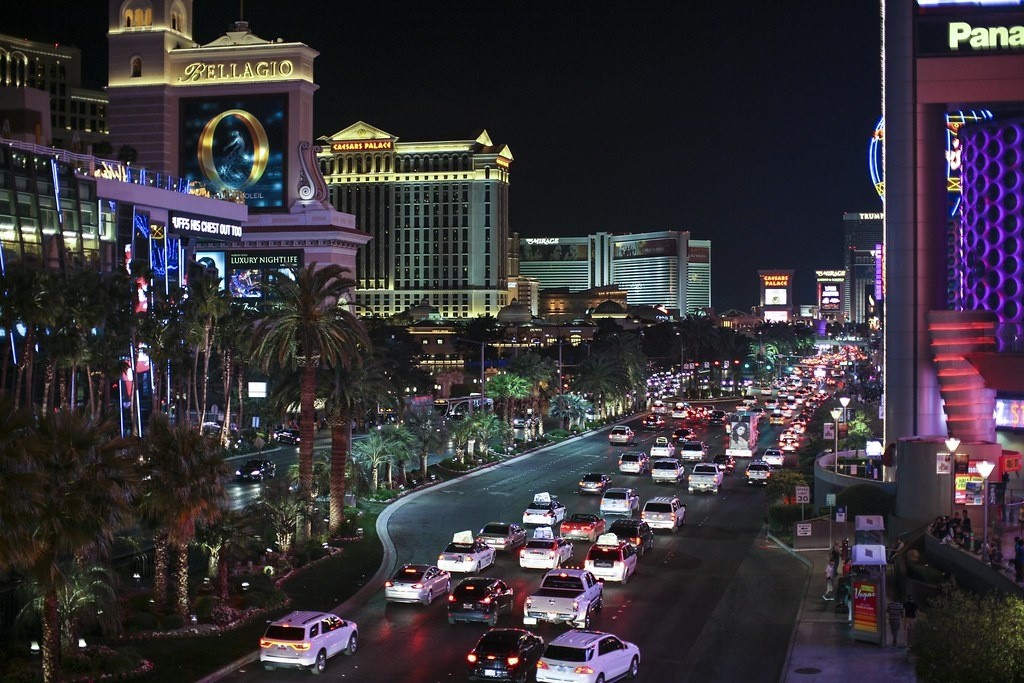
274 428 300 446
519 527 575 571
465 627 545 683
639 497 688 533
447 577 514 628
579 472 613 496
600 487 640 518
476 522 528 554
385 563 451 606
514 411 540 429
562 342 866 468
605 519 654 557
236 460 277 483
436 530 497 577
522 492 568 529
557 512 606 547
584 533 639 585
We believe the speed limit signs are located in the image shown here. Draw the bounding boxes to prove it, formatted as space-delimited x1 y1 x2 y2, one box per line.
796 486 809 503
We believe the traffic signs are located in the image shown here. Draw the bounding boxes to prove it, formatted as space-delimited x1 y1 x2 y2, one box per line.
796 524 812 536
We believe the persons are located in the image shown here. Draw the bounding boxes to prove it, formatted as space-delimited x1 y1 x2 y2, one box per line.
733 422 748 447
887 596 904 648
1018 508 1024 535
867 459 875 478
981 535 1024 581
317 419 322 431
843 558 852 577
903 595 920 646
822 560 836 600
352 420 356 435
929 509 981 552
833 546 840 575
892 538 905 552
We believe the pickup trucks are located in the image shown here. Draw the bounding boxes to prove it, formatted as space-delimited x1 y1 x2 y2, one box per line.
744 460 773 488
688 462 725 495
523 568 605 631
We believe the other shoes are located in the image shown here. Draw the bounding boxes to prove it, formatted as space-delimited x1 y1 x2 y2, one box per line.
892 645 898 648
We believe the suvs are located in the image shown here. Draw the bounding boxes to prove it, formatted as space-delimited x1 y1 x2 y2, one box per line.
652 458 685 486
618 450 650 476
259 611 360 676
536 627 640 683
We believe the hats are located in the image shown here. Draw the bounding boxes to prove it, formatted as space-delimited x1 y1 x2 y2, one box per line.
908 595 913 600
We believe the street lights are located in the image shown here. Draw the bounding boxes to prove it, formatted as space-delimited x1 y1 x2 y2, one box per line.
976 461 996 562
830 410 843 472
839 397 851 424
943 437 962 520
